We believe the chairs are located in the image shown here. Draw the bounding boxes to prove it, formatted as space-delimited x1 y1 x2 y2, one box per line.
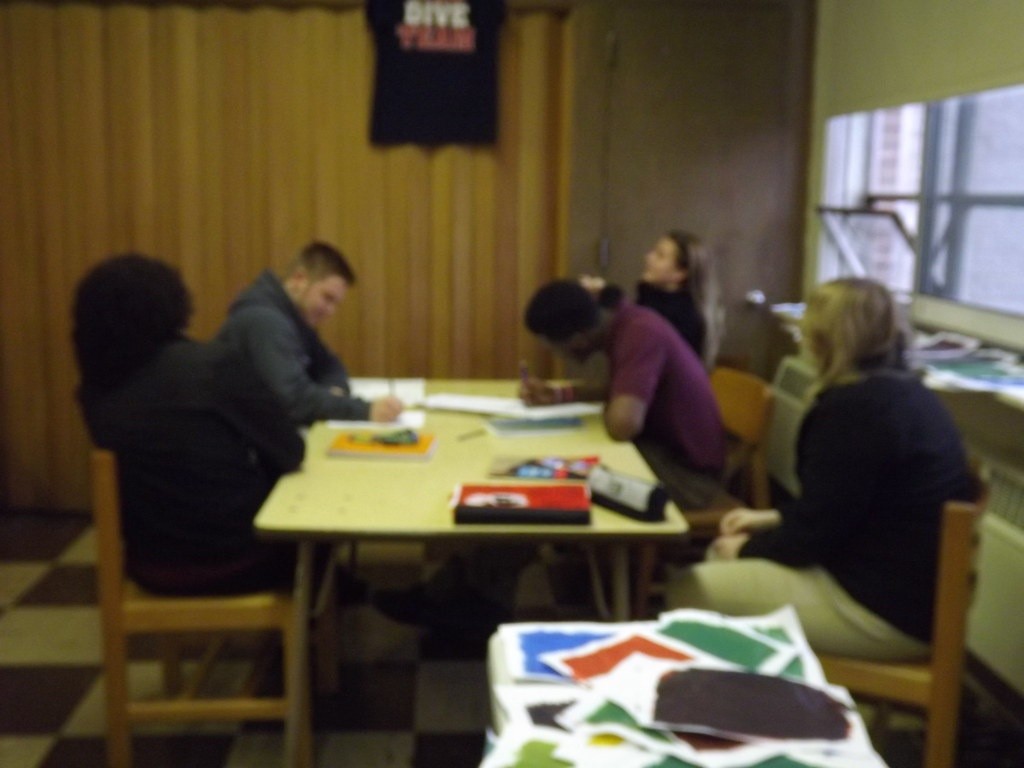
639 365 777 622
69 253 199 590
90 449 315 768
815 443 994 768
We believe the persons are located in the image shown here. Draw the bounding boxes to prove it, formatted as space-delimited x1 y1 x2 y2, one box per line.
71 251 361 732
564 230 720 386
368 277 726 660
671 279 991 663
209 242 403 595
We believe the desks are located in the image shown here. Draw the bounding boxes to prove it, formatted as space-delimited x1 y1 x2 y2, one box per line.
489 629 889 768
251 378 690 768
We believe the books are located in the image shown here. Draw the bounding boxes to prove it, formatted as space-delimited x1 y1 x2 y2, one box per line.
483 414 583 437
896 328 1024 397
452 454 673 525
329 427 433 460
